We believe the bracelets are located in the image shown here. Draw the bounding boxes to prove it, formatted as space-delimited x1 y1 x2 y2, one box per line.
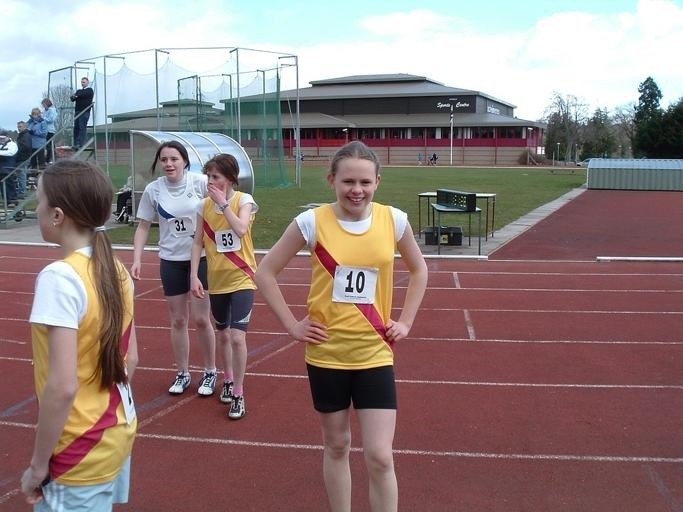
219 201 229 211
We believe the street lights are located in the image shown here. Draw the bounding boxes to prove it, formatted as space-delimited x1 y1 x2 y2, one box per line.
618 145 621 159
628 147 632 159
555 143 560 167
574 143 578 166
526 127 533 166
450 105 454 166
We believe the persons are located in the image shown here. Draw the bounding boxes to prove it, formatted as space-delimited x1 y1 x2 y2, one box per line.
19 158 137 512
190 154 258 420
111 165 143 222
69 77 93 151
130 141 217 396
0 133 18 205
415 152 440 167
13 121 32 199
40 98 58 164
255 140 429 511
25 108 47 181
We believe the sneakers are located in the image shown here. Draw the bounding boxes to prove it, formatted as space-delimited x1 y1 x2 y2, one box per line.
169 372 246 420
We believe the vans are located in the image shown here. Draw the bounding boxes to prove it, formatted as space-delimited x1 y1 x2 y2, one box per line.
576 158 590 166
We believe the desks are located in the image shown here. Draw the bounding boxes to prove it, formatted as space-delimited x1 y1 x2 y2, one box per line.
430 203 482 255
417 191 496 242
